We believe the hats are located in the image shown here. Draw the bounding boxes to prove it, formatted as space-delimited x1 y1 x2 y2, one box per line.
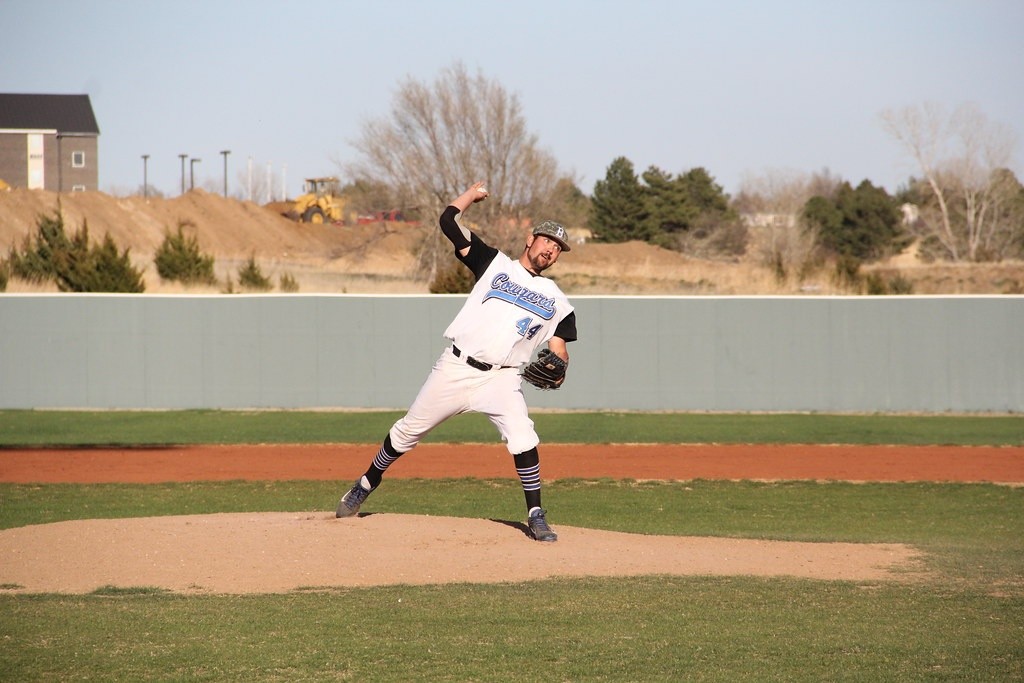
532 220 570 252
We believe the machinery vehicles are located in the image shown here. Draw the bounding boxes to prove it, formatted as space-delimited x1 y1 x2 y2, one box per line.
280 177 359 226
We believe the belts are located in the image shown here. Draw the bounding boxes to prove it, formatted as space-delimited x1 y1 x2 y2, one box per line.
452 345 511 371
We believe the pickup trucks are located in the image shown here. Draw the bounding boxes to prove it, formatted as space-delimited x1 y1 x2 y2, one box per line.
357 209 422 226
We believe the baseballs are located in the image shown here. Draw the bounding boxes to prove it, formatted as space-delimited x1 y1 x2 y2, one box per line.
478 187 487 201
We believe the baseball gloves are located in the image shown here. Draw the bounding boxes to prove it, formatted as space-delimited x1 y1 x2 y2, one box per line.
516 348 568 391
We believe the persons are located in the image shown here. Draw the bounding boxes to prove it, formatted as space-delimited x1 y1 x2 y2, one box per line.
335 182 578 541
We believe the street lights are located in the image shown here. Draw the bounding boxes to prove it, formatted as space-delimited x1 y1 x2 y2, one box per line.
140 155 149 198
220 151 231 198
178 154 188 195
191 159 201 191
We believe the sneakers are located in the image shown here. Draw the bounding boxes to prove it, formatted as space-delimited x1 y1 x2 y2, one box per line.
336 473 382 518
528 509 557 542
